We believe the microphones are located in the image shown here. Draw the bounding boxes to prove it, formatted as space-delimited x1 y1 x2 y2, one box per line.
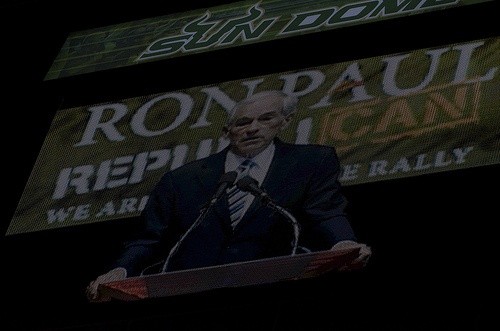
161 171 237 272
236 176 300 256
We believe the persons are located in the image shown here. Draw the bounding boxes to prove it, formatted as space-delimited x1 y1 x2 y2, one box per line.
84 90 373 303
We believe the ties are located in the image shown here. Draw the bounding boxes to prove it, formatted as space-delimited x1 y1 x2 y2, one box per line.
227 159 255 231
